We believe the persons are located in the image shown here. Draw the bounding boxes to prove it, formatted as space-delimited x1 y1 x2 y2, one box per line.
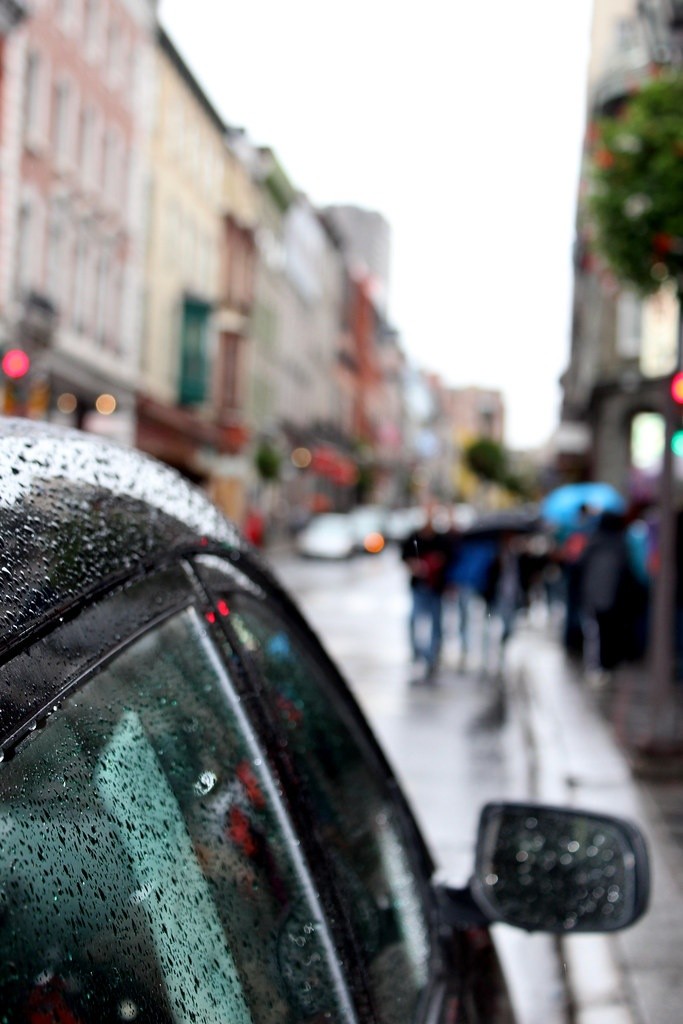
195 772 297 927
400 501 630 714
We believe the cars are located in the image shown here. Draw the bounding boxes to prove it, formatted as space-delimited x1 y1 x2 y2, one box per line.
0 416 652 1024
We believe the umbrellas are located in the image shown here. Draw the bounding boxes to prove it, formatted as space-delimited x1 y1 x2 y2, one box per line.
540 478 628 530
441 501 543 535
